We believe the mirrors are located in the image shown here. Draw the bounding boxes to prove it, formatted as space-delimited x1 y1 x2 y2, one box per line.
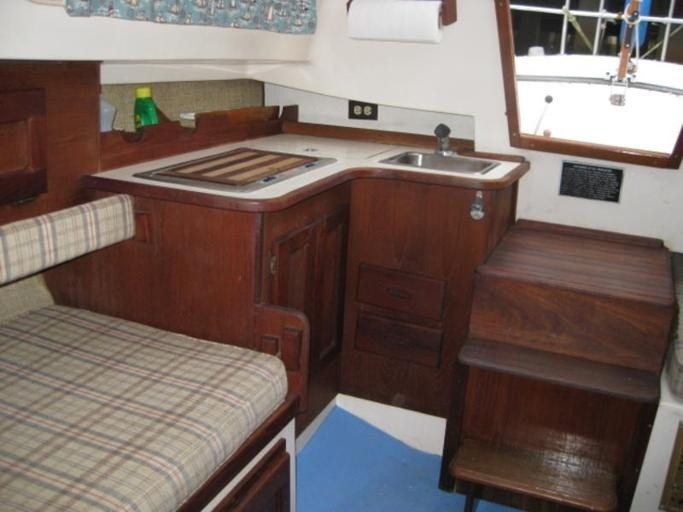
494 0 682 169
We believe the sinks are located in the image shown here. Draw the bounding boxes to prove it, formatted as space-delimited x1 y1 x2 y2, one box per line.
377 150 502 175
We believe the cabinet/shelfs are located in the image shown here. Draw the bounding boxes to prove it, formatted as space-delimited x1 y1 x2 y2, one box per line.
89 171 351 413
350 168 520 423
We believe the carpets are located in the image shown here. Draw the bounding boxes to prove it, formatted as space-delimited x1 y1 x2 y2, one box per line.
296 407 521 511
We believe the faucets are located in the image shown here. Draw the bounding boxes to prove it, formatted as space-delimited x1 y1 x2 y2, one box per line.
433 123 452 154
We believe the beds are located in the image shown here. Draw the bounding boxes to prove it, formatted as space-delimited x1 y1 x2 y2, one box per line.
0 193 309 512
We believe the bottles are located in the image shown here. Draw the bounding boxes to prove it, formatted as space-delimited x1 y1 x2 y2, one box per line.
99 94 115 133
